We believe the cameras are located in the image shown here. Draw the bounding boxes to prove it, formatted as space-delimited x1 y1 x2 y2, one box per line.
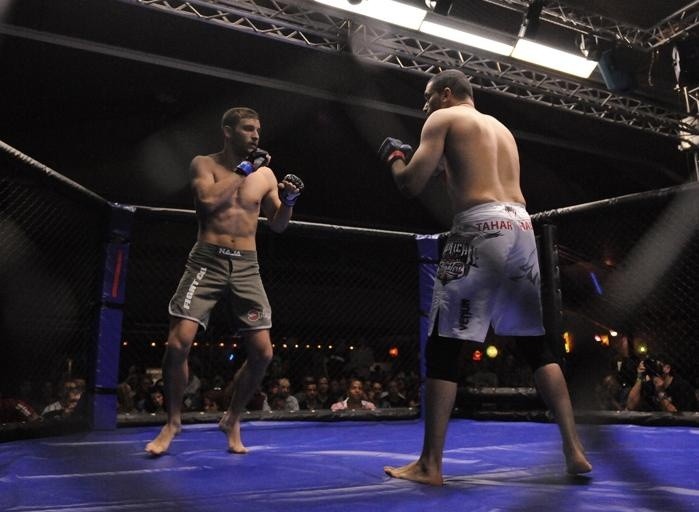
642 358 664 377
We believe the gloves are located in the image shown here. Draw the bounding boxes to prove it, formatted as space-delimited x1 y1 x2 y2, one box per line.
375 136 414 178
232 147 273 177
276 173 305 208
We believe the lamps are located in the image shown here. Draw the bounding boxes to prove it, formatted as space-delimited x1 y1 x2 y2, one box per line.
316 0 600 81
580 32 638 95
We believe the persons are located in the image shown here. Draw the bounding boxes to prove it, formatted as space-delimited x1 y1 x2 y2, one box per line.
142 106 306 458
374 69 592 487
0 331 699 425
